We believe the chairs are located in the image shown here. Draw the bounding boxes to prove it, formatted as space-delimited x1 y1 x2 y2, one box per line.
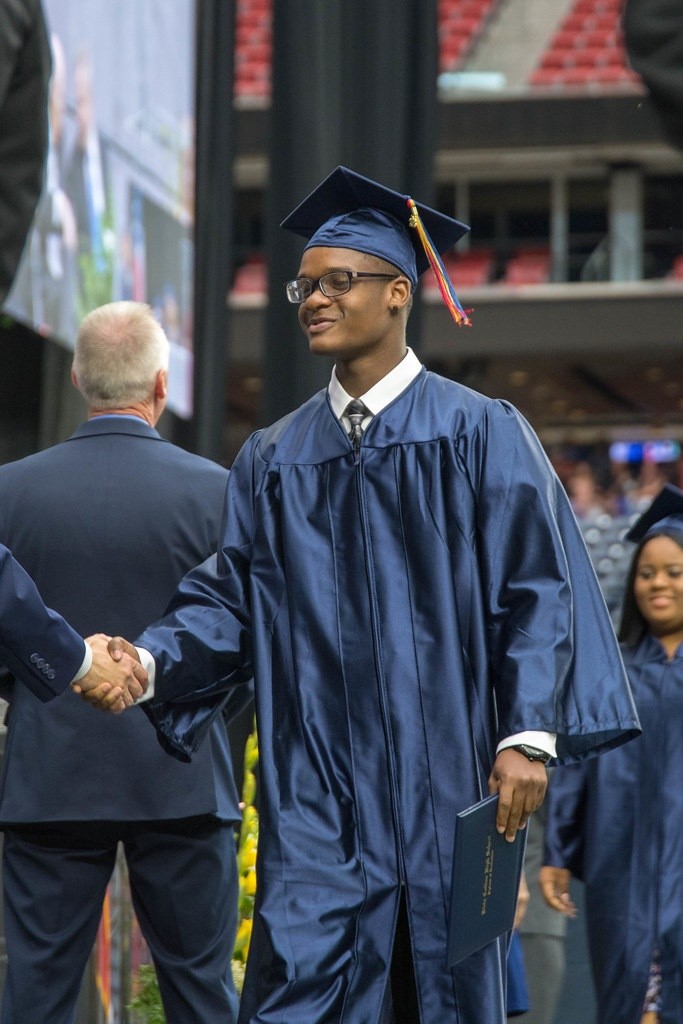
528 0 638 84
437 0 496 72
230 245 548 291
233 0 272 96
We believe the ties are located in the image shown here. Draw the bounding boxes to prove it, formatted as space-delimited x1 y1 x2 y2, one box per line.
344 398 371 453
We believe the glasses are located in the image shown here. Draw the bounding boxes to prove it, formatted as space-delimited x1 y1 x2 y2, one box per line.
282 271 399 304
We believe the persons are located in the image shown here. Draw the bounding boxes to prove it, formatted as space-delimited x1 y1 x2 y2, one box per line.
538 480 682 1023
0 542 154 718
553 446 670 521
0 297 256 1024
12 33 188 354
65 167 648 1024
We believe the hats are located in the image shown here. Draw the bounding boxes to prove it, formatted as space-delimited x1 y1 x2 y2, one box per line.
279 166 475 329
625 482 683 538
